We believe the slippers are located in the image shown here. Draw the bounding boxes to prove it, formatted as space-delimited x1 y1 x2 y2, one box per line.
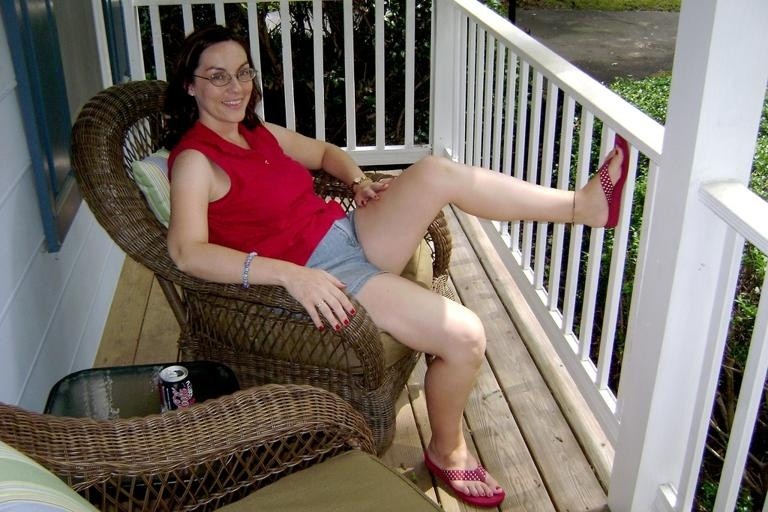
595 131 631 231
424 439 506 509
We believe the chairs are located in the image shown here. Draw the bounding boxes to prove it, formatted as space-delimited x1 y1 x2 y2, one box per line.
67 79 458 458
0 381 446 512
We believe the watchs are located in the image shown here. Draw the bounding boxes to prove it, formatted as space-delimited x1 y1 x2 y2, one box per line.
350 176 371 191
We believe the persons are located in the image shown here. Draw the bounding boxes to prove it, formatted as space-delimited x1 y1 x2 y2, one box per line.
161 25 630 507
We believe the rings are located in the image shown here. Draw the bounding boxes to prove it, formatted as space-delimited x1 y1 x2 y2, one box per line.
316 301 324 308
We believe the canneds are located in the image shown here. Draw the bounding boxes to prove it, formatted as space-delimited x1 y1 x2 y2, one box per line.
158 365 197 413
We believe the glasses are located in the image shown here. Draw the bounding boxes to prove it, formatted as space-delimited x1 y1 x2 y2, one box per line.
190 67 258 88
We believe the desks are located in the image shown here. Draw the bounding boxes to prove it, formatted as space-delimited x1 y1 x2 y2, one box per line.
42 359 247 500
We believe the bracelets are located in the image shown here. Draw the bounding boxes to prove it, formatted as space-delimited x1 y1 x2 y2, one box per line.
243 252 257 289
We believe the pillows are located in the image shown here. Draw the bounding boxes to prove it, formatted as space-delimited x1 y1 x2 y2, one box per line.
0 435 104 512
130 143 174 230
